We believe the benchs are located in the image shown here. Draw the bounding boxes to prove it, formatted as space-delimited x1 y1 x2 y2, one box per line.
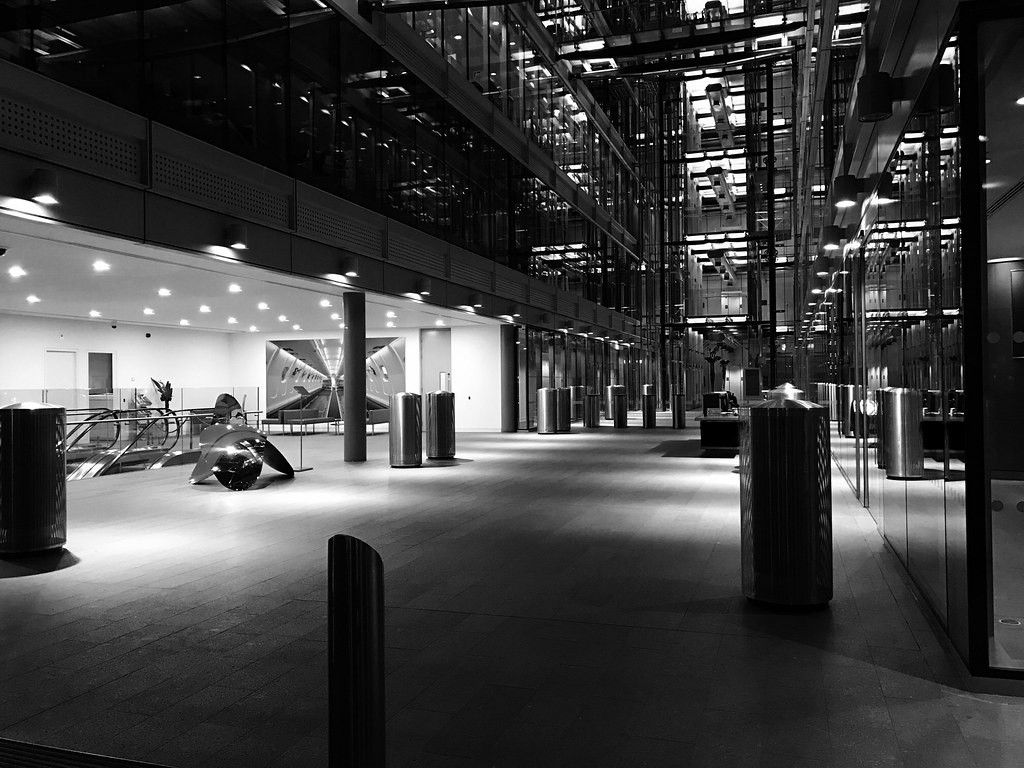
263 409 334 434
366 409 389 436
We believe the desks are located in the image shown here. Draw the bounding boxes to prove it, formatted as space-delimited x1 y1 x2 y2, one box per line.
335 418 344 435
